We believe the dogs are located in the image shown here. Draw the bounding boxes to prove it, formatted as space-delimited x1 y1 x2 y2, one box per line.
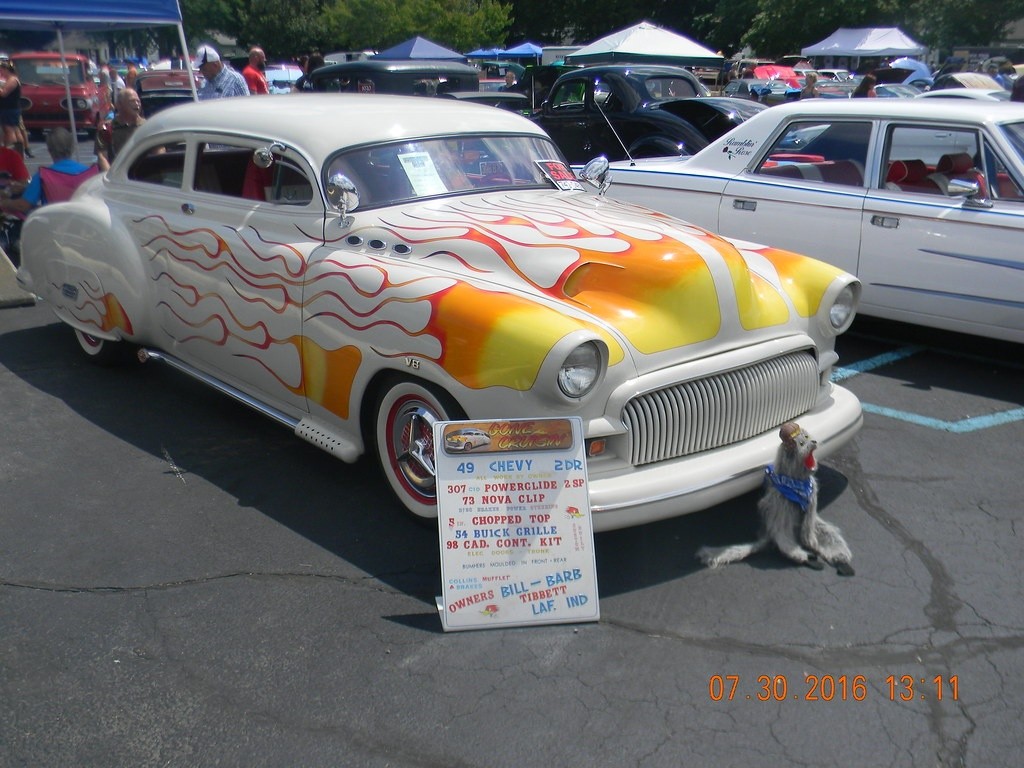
692 424 853 569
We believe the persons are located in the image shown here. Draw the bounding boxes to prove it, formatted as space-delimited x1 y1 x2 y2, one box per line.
931 61 936 74
97 60 137 107
243 48 269 96
498 72 515 92
194 46 249 99
988 64 1004 86
292 56 325 93
851 74 877 97
800 73 819 98
94 89 144 172
1011 75 1024 102
0 64 89 257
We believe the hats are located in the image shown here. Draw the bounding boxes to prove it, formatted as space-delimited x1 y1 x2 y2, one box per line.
192 45 219 69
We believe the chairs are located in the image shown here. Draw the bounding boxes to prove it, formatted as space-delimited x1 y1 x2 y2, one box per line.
884 152 977 197
0 146 100 266
243 159 276 203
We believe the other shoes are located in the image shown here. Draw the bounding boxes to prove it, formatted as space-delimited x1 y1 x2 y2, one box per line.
24 148 35 158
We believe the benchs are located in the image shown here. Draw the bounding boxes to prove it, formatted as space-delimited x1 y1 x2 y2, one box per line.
760 157 866 187
143 150 249 191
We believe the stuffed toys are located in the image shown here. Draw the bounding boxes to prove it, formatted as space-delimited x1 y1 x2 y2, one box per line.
699 424 855 576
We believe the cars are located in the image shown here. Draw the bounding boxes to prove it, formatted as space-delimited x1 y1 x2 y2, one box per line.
10 52 112 137
584 98 1024 343
16 89 865 531
494 64 771 165
700 59 1024 169
437 75 517 91
265 63 309 93
305 59 529 109
125 66 204 112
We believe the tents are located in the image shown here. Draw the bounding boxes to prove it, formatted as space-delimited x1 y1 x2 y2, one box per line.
465 42 542 66
370 36 466 63
0 0 199 160
801 28 928 68
564 23 724 96
